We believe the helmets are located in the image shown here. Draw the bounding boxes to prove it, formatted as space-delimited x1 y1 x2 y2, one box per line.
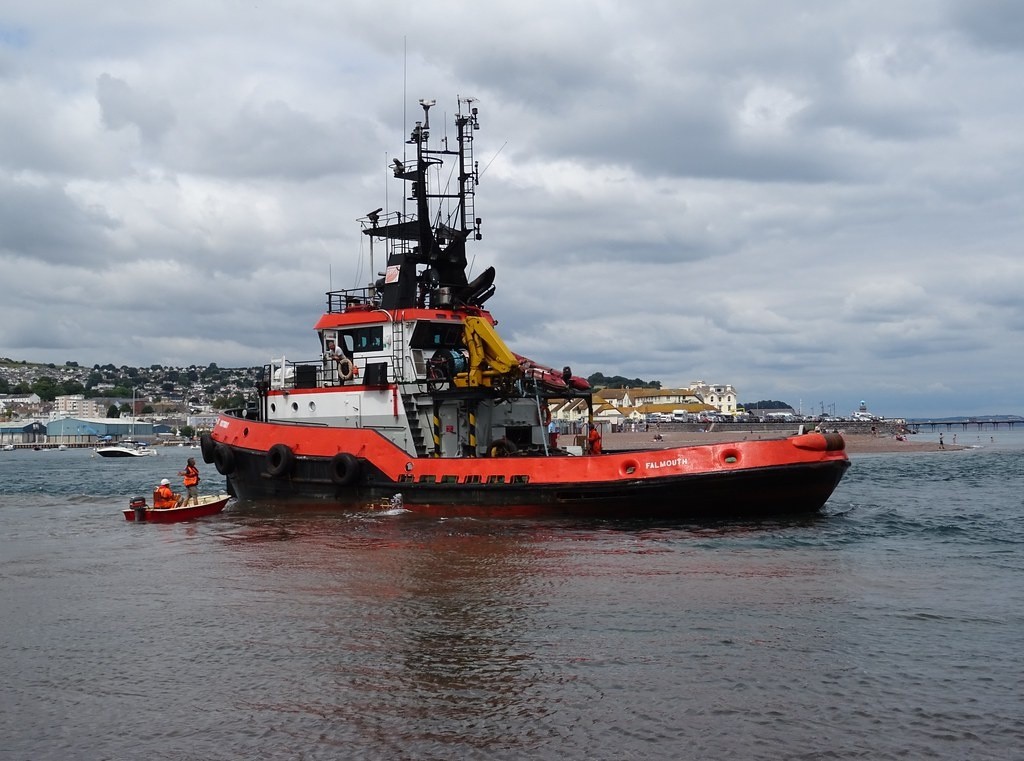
160 478 171 486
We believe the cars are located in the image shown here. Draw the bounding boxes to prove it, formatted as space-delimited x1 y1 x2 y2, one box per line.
622 404 860 427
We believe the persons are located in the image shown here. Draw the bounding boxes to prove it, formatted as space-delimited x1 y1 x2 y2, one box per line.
620 413 927 448
939 433 944 450
953 433 956 444
976 435 980 443
154 478 181 509
329 341 356 374
547 418 557 443
586 424 602 457
176 458 202 506
990 436 994 444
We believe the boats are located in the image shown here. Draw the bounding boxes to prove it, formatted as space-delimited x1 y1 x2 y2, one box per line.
4 412 200 457
123 494 233 524
199 44 853 524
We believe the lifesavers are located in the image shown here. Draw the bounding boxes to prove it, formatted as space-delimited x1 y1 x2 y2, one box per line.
337 359 353 380
